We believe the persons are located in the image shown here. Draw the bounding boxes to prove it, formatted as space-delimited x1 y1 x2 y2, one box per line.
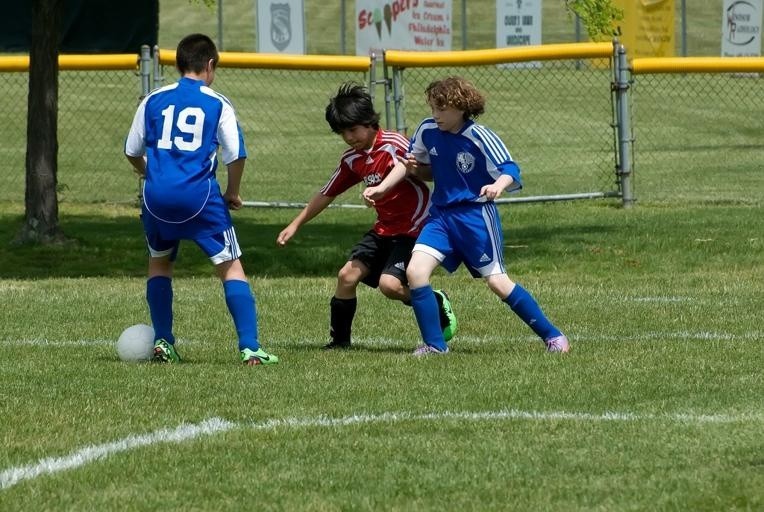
124 33 279 366
277 78 457 357
362 79 571 356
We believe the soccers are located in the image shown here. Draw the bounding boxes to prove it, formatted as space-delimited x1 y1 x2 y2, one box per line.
117 324 155 360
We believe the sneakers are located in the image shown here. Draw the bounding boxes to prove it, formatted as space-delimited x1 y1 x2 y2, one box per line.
544 332 569 353
152 337 180 363
321 342 352 349
414 288 457 354
241 347 279 367
